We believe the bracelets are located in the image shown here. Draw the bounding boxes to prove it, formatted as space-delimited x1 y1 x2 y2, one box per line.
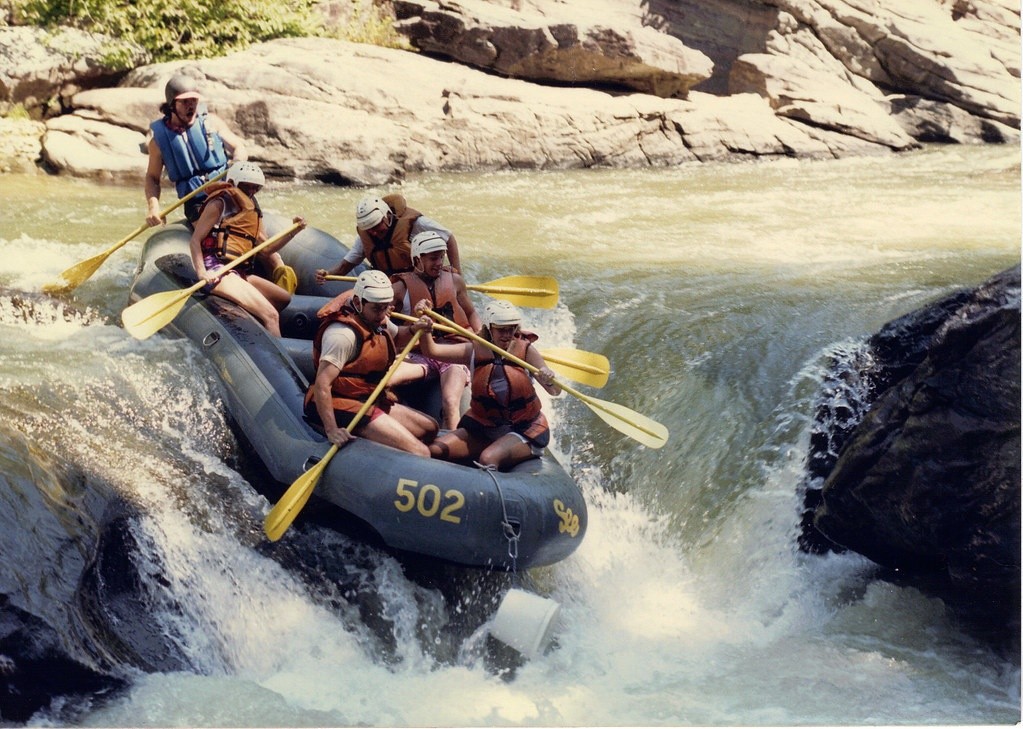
408 324 415 336
290 232 294 237
546 384 553 386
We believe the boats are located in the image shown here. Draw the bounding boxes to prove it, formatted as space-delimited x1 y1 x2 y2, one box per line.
128 206 587 571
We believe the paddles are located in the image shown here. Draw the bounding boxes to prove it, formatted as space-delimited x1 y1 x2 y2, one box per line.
262 318 428 544
417 299 669 450
121 214 306 341
44 167 231 292
319 269 560 309
389 311 611 389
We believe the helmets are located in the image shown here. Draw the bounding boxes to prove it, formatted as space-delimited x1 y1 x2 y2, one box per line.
165 75 199 104
410 231 448 272
485 300 521 331
225 161 265 189
356 196 393 230
353 269 394 313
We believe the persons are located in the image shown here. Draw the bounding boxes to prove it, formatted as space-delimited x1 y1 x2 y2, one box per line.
302 270 439 458
189 160 307 338
386 230 483 431
144 76 249 231
414 299 562 473
315 193 462 285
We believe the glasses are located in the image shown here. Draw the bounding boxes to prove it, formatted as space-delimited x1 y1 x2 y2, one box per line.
241 185 258 190
